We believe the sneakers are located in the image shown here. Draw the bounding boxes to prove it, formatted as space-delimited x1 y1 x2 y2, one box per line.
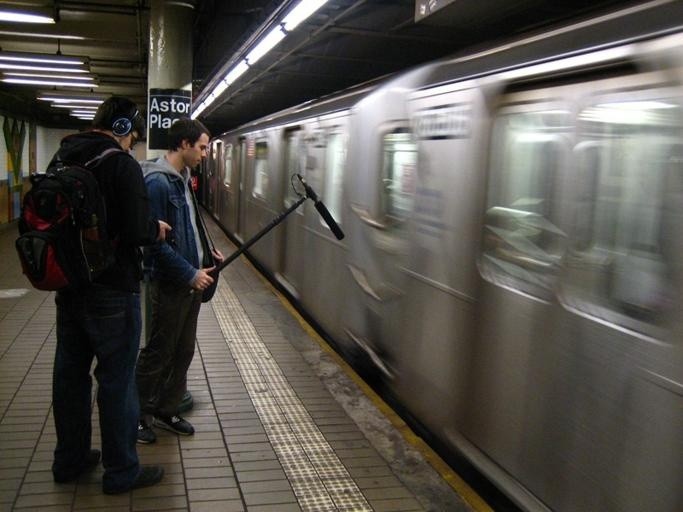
104 467 163 493
137 419 155 444
81 449 100 476
152 410 195 435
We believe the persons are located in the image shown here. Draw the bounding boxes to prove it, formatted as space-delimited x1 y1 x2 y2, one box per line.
136 118 224 444
45 95 165 494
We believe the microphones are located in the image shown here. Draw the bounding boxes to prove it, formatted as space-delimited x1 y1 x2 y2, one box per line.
298 173 346 241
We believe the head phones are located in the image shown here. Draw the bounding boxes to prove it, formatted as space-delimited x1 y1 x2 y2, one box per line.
113 107 140 138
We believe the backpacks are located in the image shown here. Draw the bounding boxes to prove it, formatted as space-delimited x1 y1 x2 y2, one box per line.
16 148 128 291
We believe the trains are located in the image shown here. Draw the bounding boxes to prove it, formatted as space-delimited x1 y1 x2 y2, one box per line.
198 0 683 512
